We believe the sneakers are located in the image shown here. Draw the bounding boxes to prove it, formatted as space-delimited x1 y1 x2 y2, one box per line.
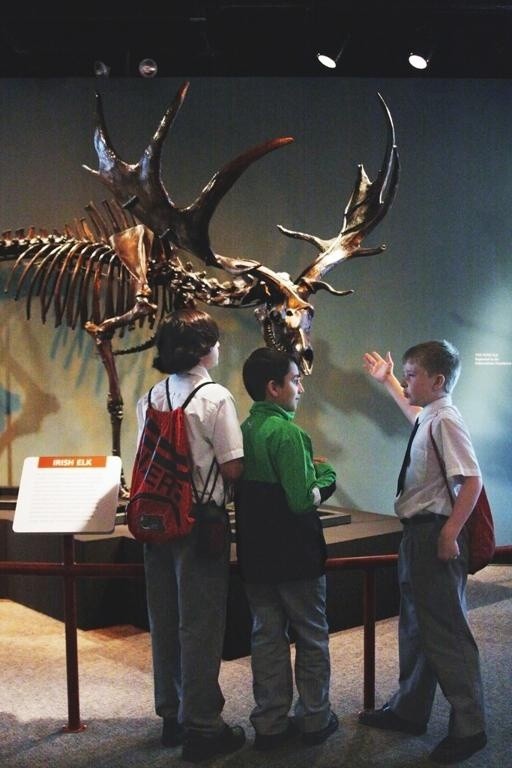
253 715 303 751
357 700 429 737
301 708 340 745
158 714 189 747
427 729 488 765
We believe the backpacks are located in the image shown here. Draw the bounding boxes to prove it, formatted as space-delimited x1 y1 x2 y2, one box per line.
124 380 220 546
428 404 498 577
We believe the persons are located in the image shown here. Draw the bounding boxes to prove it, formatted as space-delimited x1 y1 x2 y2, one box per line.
353 333 489 766
126 314 247 767
230 340 340 753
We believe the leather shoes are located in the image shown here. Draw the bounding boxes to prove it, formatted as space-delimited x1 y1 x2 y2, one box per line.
181 721 248 762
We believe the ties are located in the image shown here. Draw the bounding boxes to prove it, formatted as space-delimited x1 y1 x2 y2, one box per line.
394 415 422 498
